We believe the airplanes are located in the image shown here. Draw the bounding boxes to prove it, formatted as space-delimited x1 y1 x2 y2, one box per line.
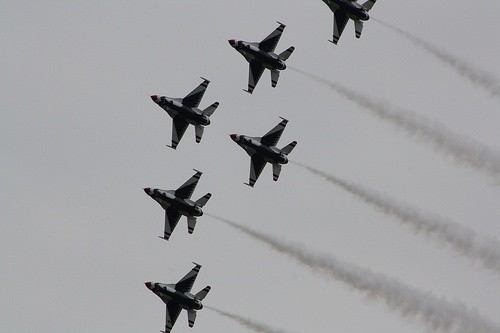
144 168 212 241
150 76 219 150
229 21 296 94
230 115 298 187
323 0 377 45
145 261 211 333
